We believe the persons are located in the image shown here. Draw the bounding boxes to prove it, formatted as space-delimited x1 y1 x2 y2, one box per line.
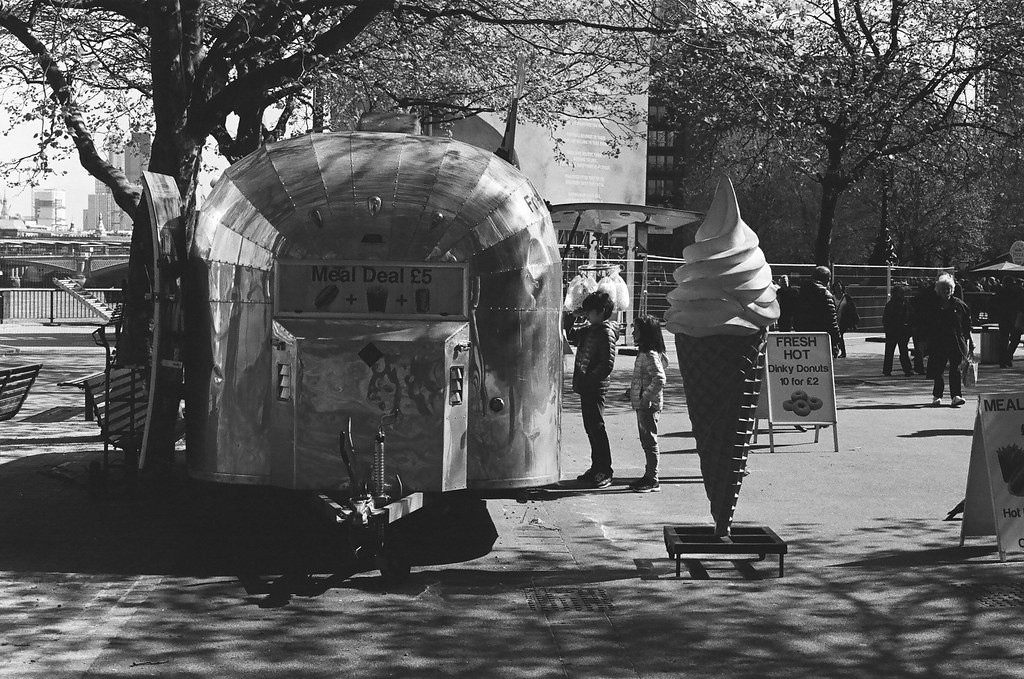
881 285 914 377
775 274 800 332
564 291 619 487
954 273 1024 367
630 314 669 493
793 265 842 358
831 279 859 358
931 275 976 406
912 281 936 378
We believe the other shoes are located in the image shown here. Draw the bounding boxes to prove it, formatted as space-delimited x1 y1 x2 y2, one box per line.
904 371 912 377
884 373 890 376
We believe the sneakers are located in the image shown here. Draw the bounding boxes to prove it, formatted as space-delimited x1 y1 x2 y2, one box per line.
629 476 660 492
932 396 940 405
952 395 965 406
574 467 614 488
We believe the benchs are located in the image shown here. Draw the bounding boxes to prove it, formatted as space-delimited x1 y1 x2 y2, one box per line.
0 364 43 421
86 369 149 480
57 372 104 419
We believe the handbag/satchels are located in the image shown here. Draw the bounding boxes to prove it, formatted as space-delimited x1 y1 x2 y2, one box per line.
961 352 978 387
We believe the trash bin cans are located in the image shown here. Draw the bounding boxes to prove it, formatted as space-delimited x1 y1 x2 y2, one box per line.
978 323 1005 362
